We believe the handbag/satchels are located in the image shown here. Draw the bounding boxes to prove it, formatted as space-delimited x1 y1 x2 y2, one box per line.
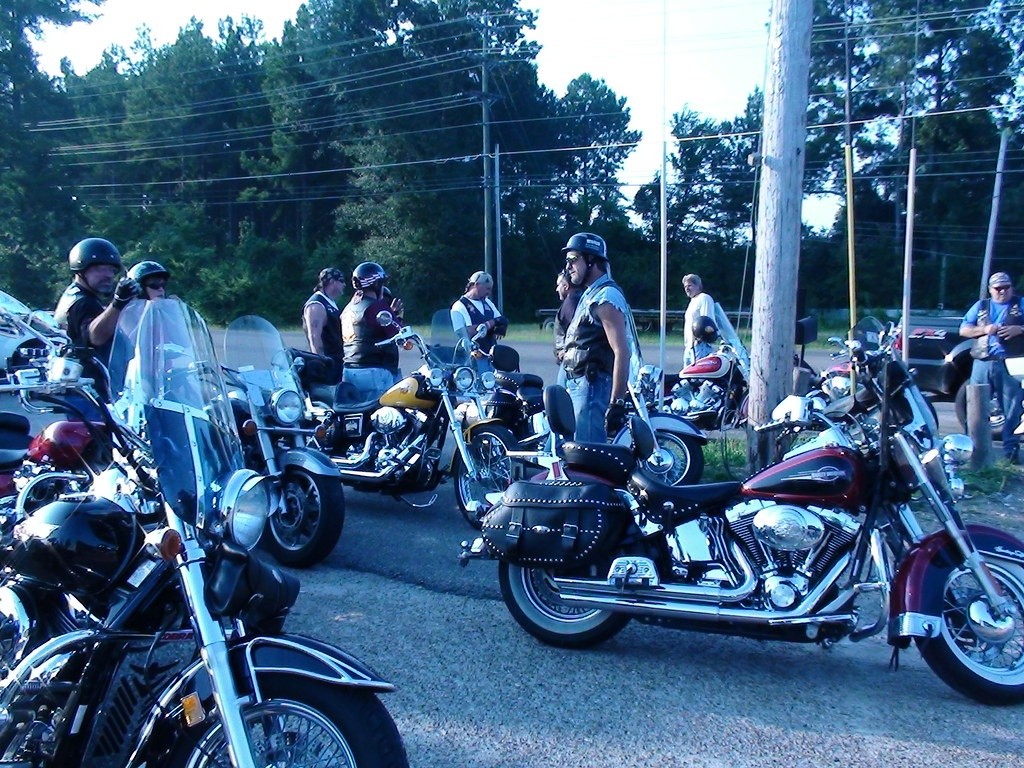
479 481 626 569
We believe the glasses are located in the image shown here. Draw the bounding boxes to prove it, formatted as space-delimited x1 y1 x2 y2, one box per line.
994 285 1010 291
338 279 346 284
565 256 578 264
146 283 165 290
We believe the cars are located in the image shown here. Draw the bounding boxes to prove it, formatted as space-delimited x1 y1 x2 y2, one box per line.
0 284 67 382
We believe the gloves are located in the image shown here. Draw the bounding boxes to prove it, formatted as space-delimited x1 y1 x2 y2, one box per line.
112 277 142 311
390 298 403 315
604 404 625 436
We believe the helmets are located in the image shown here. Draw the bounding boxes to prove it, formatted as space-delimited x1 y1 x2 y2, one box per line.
352 262 385 289
381 286 392 298
22 421 98 470
561 233 609 262
692 316 718 343
128 261 170 283
69 238 121 271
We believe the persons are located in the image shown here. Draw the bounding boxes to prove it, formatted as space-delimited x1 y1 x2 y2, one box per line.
450 272 509 374
302 268 346 365
340 263 404 401
54 238 192 402
959 272 1024 469
682 274 717 369
543 232 633 454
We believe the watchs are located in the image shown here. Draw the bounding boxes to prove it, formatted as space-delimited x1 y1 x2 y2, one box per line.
610 399 625 406
1020 325 1024 333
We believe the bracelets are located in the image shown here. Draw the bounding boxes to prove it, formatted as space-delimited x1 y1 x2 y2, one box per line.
486 321 491 330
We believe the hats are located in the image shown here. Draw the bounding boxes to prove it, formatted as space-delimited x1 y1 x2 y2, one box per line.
989 272 1011 287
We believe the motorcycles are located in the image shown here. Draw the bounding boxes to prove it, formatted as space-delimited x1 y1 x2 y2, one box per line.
225 285 545 564
460 303 1024 714
2 293 421 768
